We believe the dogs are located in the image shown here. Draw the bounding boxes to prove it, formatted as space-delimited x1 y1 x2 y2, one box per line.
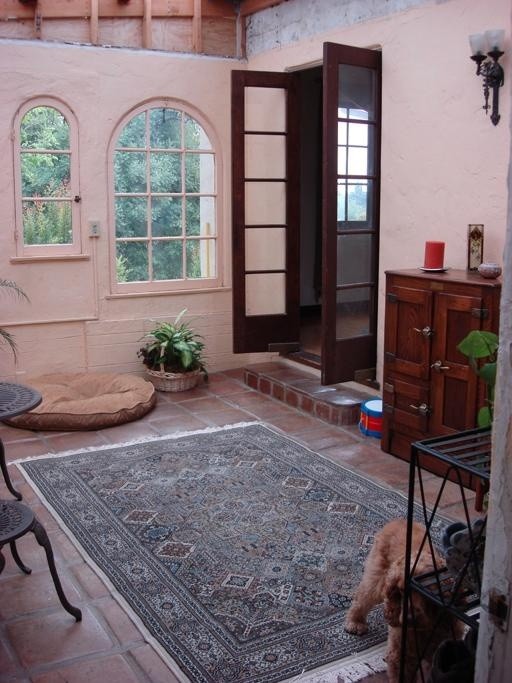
342 519 466 683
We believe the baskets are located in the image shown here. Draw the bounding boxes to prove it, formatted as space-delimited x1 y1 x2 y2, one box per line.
146 347 200 392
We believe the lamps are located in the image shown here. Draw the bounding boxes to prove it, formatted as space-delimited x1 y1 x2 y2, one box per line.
468 28 507 126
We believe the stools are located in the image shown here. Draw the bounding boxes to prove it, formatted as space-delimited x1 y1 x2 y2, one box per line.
1 499 82 624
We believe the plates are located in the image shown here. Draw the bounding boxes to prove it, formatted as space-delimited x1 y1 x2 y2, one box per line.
418 267 451 272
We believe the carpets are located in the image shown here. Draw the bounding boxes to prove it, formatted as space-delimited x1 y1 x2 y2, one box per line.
12 418 481 682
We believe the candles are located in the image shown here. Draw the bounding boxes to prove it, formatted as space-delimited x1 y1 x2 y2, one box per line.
424 242 445 268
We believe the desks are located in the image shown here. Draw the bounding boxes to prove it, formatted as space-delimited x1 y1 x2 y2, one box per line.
0 382 43 500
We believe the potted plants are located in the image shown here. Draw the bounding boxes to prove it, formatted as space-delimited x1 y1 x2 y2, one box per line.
136 309 211 392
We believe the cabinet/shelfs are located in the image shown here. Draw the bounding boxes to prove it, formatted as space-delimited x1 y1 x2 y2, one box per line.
380 267 502 496
